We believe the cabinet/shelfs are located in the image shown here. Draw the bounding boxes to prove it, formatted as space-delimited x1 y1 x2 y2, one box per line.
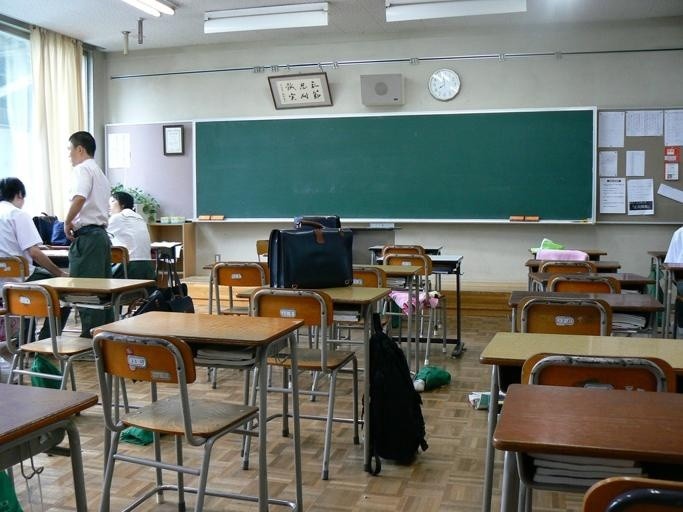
147 223 196 278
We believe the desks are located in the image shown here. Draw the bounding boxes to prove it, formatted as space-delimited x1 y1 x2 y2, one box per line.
351 263 421 378
492 384 683 512
0 383 99 512
508 291 665 338
375 254 464 358
151 242 182 288
91 310 302 512
369 245 443 328
203 262 243 383
342 227 402 264
648 251 667 326
525 259 621 292
661 263 683 338
527 273 656 294
235 288 392 472
479 332 683 512
25 277 156 416
31 250 69 268
528 249 608 261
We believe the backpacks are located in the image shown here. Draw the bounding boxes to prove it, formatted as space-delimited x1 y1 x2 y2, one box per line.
358 314 430 476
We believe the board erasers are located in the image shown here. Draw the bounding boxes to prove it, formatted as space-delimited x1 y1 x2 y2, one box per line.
525 216 540 222
199 215 210 220
509 215 525 221
211 215 224 220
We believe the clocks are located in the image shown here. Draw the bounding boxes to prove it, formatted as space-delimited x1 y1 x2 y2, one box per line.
429 66 461 102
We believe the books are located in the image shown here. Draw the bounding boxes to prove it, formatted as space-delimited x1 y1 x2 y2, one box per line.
193 350 256 369
59 293 110 312
527 453 644 487
609 309 648 336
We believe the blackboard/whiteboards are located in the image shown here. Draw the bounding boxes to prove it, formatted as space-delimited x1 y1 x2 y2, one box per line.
193 106 597 225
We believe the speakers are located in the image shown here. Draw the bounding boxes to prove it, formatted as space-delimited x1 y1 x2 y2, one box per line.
359 73 405 107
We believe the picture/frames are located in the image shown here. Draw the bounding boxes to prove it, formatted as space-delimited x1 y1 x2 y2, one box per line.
268 72 333 110
162 126 184 156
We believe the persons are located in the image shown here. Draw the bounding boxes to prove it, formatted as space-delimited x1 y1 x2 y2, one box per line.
658 222 683 301
0 179 69 359
64 129 112 332
67 191 151 287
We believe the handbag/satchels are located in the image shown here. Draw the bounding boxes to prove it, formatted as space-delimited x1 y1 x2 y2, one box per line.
268 221 353 290
51 220 71 246
33 212 58 244
128 254 194 317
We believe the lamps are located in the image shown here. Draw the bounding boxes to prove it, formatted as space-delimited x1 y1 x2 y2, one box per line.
386 1 502 21
122 0 178 17
203 3 329 33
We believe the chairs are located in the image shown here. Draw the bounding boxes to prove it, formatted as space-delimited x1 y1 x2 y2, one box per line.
517 296 613 337
110 246 149 319
94 331 259 511
583 477 683 512
539 260 597 273
382 245 439 336
49 246 70 250
212 262 272 388
241 288 361 480
0 256 35 345
380 253 451 368
236 240 269 298
2 284 119 425
520 353 678 394
310 268 386 401
546 276 622 294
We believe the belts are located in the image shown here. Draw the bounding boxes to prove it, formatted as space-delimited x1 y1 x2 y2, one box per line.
71 226 104 238
